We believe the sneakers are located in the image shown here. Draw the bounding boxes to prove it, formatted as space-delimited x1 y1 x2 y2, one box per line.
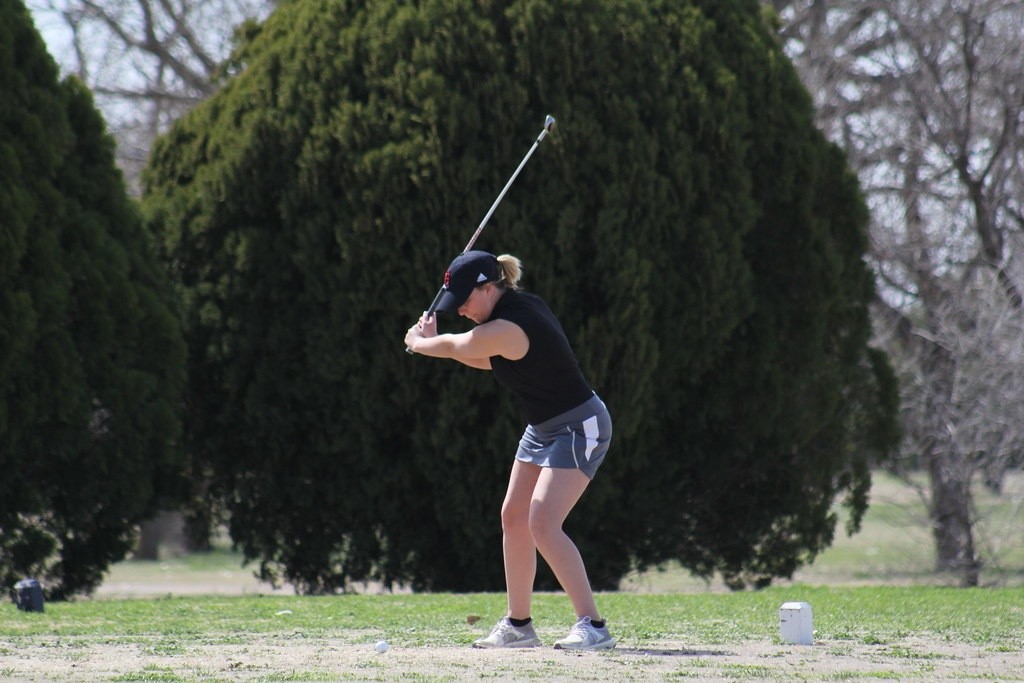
555 617 617 650
473 616 542 648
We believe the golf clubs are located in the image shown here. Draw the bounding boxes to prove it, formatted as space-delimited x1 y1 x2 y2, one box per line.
403 114 557 355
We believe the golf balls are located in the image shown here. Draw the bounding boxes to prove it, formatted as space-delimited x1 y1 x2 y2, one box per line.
376 640 388 652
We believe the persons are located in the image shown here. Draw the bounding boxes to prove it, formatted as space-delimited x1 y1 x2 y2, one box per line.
403 249 619 653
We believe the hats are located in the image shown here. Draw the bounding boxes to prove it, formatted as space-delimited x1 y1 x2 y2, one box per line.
435 250 500 312
